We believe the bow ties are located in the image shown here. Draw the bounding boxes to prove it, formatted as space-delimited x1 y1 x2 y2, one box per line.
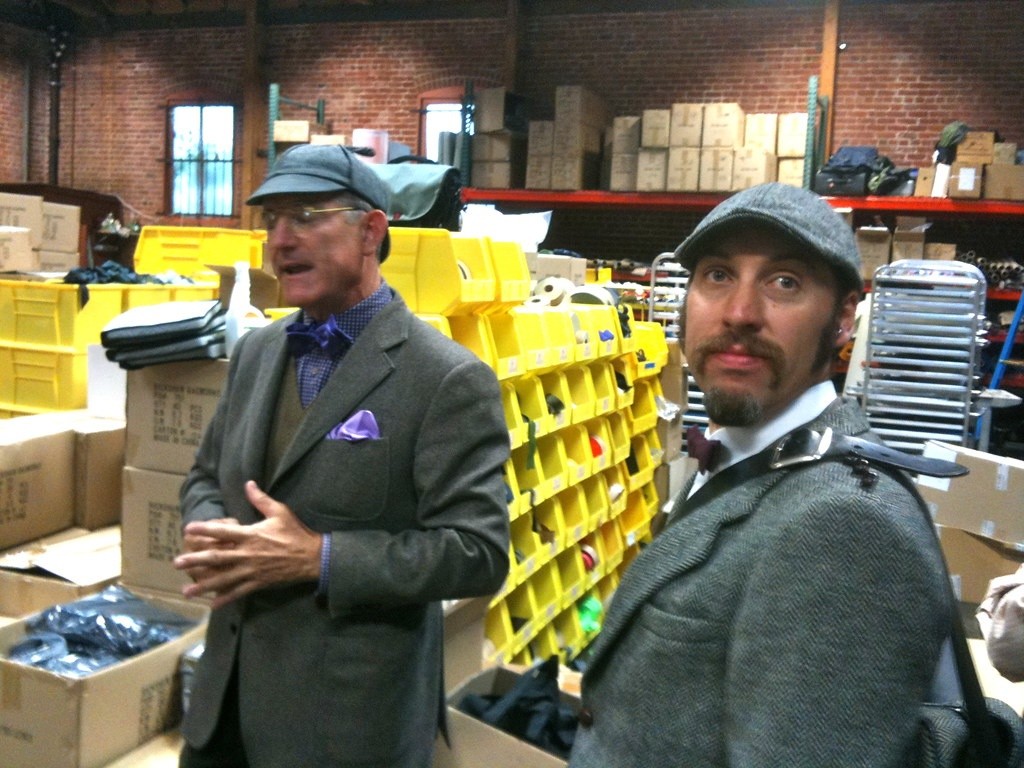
286 314 350 358
686 424 721 475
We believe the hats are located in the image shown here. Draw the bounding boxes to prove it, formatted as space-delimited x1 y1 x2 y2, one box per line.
673 183 864 293
245 144 387 213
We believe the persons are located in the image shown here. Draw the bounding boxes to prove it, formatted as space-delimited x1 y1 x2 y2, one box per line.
568 181 949 768
173 144 512 768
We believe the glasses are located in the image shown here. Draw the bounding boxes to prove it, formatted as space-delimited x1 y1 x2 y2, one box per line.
248 201 369 242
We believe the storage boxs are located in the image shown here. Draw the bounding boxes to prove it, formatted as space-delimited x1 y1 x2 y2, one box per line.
0 84 1024 768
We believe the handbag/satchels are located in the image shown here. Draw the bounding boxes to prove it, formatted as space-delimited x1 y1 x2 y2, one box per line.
364 155 466 232
902 696 1024 768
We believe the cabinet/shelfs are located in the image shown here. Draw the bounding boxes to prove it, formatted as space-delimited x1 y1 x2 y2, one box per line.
461 75 1024 448
378 226 669 666
